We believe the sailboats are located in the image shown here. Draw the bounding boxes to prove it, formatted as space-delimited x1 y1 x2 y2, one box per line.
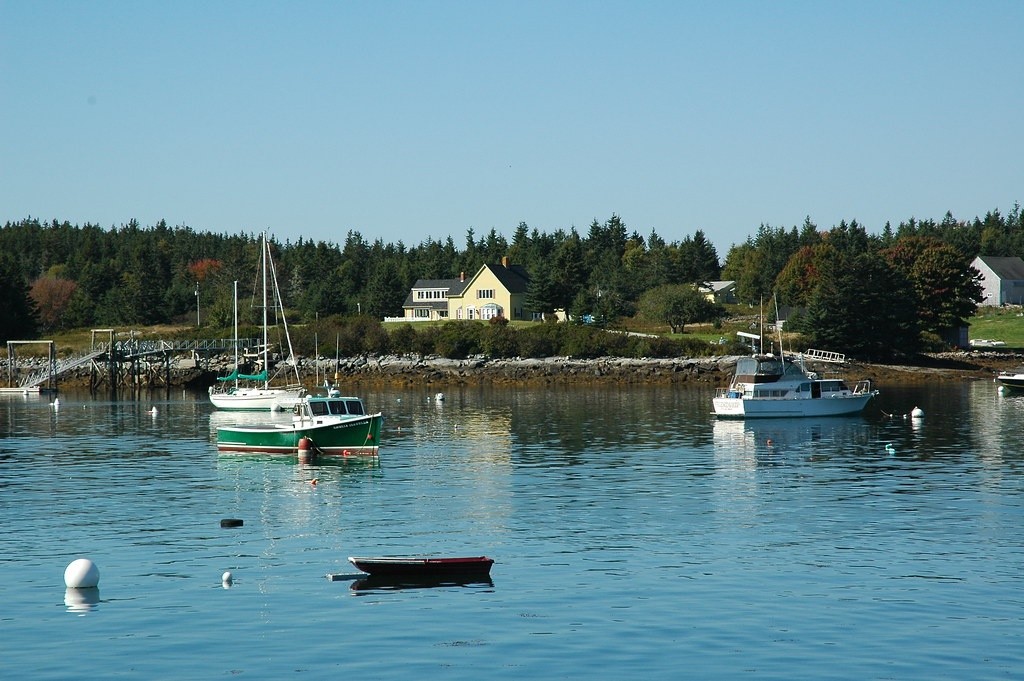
208 231 314 410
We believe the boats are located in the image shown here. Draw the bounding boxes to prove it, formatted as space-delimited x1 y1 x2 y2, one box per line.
998 370 1024 392
215 331 384 458
708 292 881 418
347 555 495 578
